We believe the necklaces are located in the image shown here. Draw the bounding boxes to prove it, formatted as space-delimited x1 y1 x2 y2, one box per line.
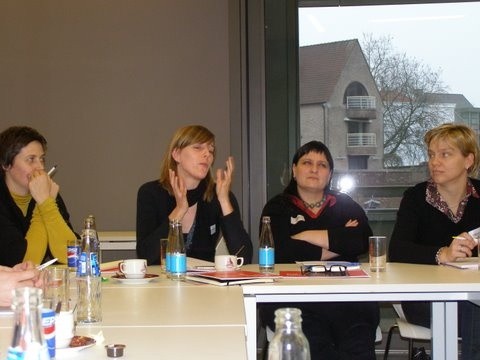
304 194 326 208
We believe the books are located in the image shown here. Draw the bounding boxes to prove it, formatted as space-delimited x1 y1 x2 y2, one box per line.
443 257 480 269
185 274 274 286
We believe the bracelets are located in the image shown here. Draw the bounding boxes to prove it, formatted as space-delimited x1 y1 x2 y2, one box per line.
435 246 445 266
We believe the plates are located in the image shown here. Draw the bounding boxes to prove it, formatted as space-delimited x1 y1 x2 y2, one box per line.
111 274 159 284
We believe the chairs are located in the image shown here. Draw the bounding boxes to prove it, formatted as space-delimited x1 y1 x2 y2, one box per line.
382 302 463 360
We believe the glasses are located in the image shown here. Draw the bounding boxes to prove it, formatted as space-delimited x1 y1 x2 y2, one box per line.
301 264 347 276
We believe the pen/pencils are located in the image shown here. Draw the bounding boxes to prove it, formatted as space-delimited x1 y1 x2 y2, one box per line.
47 166 56 175
37 258 58 270
197 266 215 269
327 262 361 266
452 236 466 240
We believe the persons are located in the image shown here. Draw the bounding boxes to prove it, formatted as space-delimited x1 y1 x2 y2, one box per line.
136 125 255 266
258 141 381 360
387 122 480 360
0 261 49 307
0 125 81 269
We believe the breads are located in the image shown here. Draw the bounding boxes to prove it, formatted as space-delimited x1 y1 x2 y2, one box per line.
70 336 95 347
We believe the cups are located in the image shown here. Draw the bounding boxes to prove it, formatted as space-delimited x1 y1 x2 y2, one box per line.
66 240 81 278
214 254 244 272
43 267 80 311
368 236 387 272
54 309 75 348
160 239 168 277
120 259 148 278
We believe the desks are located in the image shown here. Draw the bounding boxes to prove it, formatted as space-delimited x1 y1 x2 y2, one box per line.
0 263 480 360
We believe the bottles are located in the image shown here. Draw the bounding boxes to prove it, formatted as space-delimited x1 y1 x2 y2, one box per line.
5 287 56 360
171 220 187 281
54 333 98 358
258 217 275 274
75 215 103 324
267 308 311 360
166 219 175 278
